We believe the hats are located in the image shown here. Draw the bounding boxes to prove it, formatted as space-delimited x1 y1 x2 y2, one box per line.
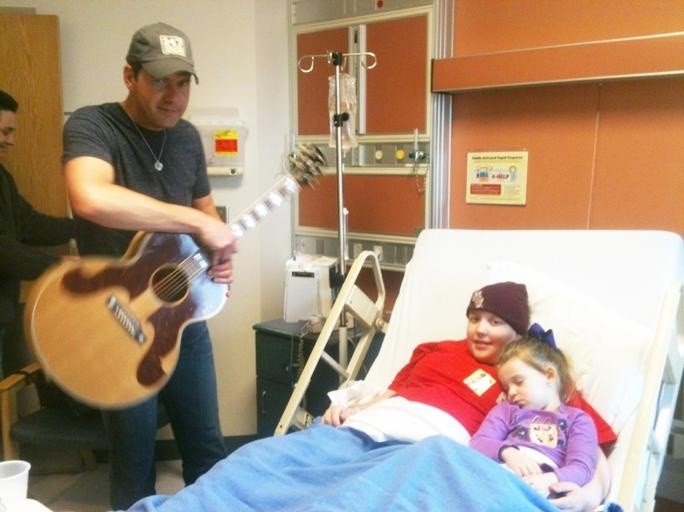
466 281 529 337
126 22 199 86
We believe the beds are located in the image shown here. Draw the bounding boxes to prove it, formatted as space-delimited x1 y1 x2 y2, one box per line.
124 228 683 511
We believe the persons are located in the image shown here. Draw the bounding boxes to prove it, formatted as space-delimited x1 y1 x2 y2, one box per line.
0 90 77 422
317 280 620 512
61 19 241 512
467 334 598 512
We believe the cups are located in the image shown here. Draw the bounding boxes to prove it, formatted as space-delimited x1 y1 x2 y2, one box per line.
0 459 32 512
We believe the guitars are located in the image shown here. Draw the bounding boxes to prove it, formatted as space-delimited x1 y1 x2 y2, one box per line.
23 141 328 410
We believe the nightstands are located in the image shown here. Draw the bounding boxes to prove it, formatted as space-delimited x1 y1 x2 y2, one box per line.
253 318 388 440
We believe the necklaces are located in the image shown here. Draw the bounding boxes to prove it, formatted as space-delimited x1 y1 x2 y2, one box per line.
127 106 168 171
